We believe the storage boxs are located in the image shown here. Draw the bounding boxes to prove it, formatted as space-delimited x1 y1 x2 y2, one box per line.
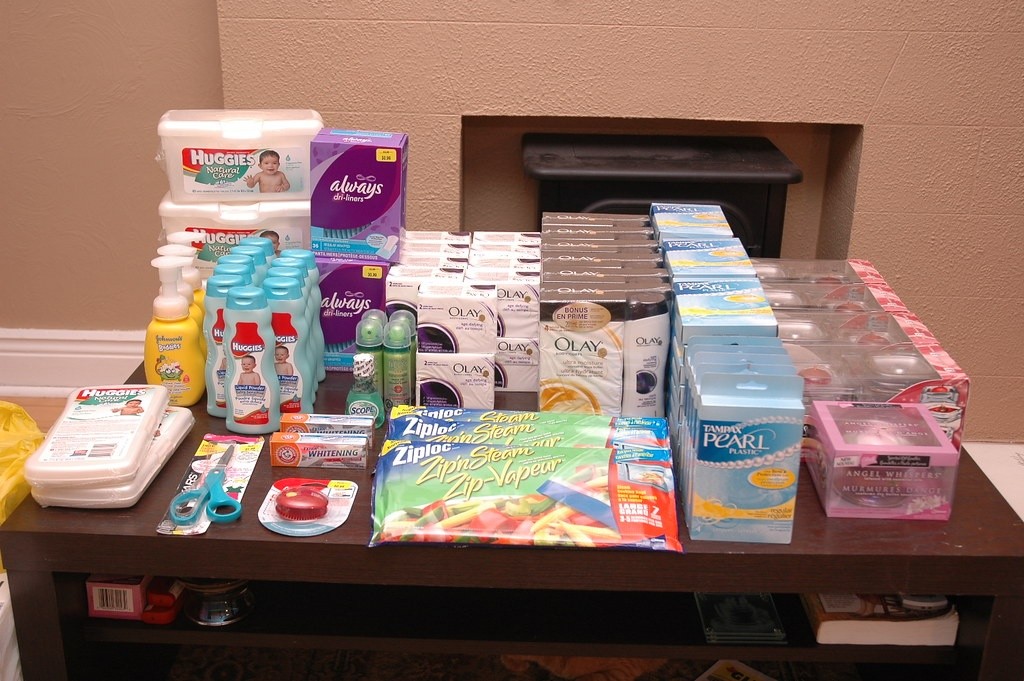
157 110 971 544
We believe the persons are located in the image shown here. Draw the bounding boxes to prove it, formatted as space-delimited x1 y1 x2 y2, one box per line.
153 423 163 440
244 150 290 259
216 345 294 396
112 399 144 415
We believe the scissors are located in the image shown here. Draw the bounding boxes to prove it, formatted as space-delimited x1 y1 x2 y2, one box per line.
168 445 242 526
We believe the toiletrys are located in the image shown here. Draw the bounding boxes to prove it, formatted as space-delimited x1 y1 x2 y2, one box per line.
205 236 327 434
621 291 670 422
141 230 207 406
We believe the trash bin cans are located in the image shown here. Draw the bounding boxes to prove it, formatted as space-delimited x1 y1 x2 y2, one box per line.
520 131 804 258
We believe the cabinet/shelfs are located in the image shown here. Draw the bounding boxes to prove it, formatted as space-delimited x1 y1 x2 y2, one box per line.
0 361 1024 681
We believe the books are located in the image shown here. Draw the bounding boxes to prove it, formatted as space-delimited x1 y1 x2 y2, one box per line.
802 588 960 645
